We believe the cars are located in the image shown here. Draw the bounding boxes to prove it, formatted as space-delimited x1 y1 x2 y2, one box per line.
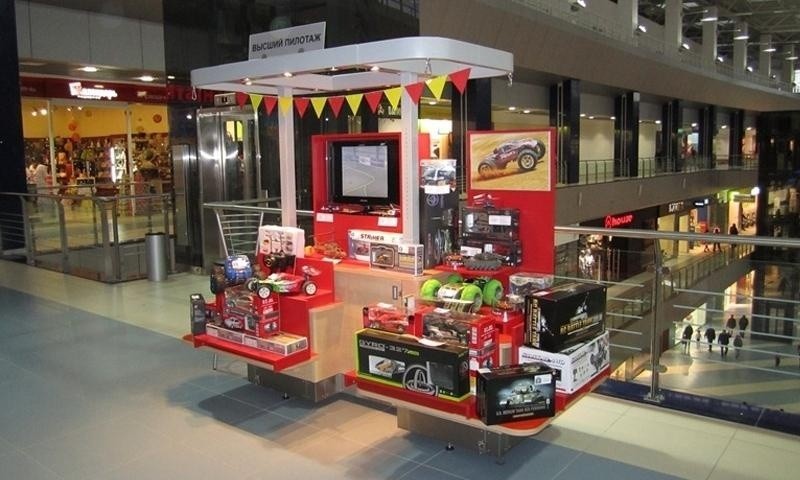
223 317 243 330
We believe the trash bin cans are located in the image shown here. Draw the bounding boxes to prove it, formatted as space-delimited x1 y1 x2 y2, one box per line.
145 232 168 282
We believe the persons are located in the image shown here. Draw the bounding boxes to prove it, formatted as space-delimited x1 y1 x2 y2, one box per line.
579 250 587 278
681 142 749 359
136 143 164 214
23 151 70 216
584 248 596 280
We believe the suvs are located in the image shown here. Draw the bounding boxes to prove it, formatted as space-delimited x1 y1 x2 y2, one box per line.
506 383 540 404
476 138 547 181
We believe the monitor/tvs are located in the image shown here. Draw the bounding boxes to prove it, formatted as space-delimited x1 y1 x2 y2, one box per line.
328 140 400 214
